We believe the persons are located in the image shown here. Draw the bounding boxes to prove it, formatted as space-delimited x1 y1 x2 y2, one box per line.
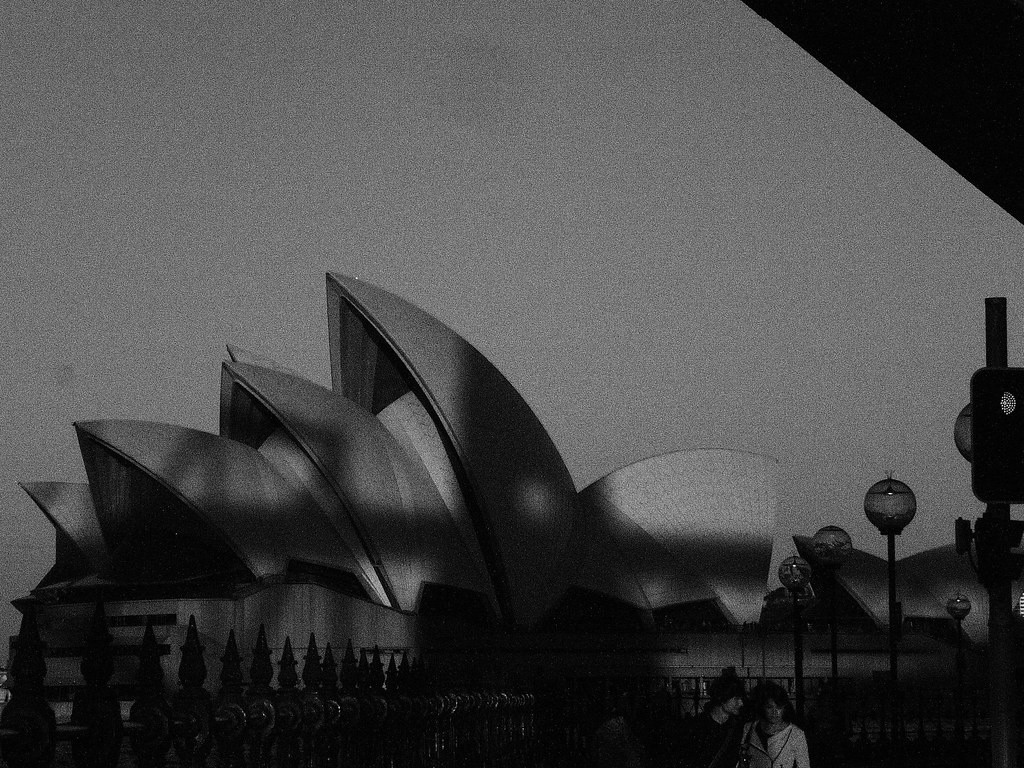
682 677 812 768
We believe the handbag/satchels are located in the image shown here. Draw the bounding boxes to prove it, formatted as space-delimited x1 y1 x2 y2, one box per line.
734 719 759 768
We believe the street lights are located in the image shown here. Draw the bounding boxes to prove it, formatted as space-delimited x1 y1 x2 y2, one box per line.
779 554 813 728
865 469 917 768
812 526 853 680
947 591 972 732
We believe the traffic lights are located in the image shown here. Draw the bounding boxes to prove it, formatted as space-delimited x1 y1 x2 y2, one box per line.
970 366 1024 502
